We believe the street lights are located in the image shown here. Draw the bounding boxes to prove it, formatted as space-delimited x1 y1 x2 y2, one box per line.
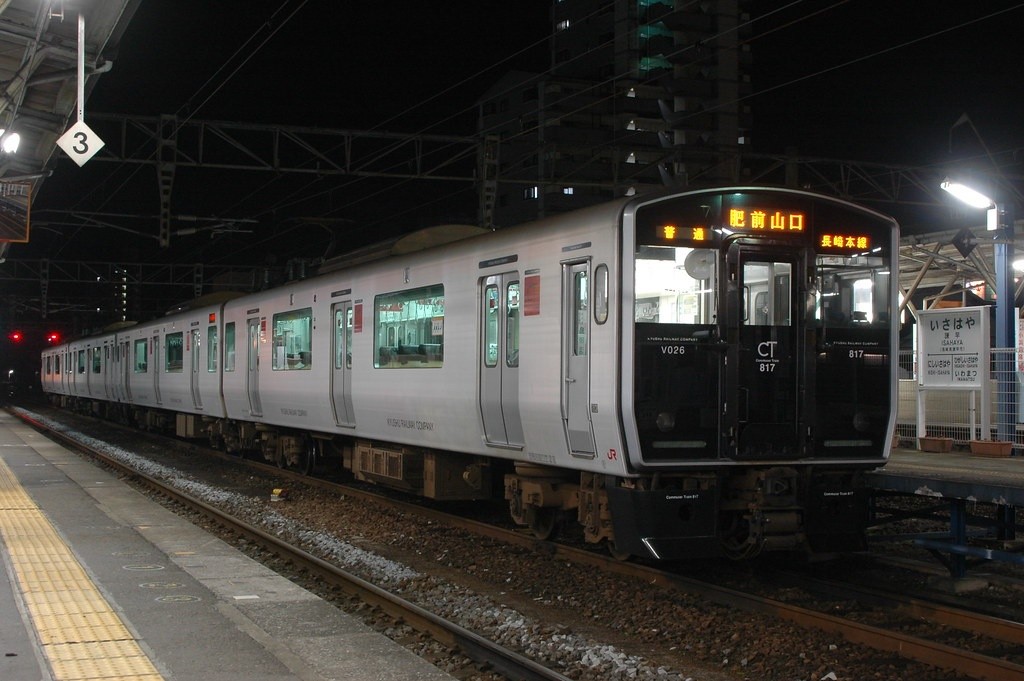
942 177 1020 441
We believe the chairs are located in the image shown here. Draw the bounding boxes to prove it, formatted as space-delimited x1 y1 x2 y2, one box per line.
419 344 440 364
298 351 311 365
398 344 420 365
378 346 397 366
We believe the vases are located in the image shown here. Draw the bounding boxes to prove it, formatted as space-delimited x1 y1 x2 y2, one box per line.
919 437 954 453
969 440 1014 459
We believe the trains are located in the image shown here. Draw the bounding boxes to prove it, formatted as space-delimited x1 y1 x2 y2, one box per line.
40 181 906 558
